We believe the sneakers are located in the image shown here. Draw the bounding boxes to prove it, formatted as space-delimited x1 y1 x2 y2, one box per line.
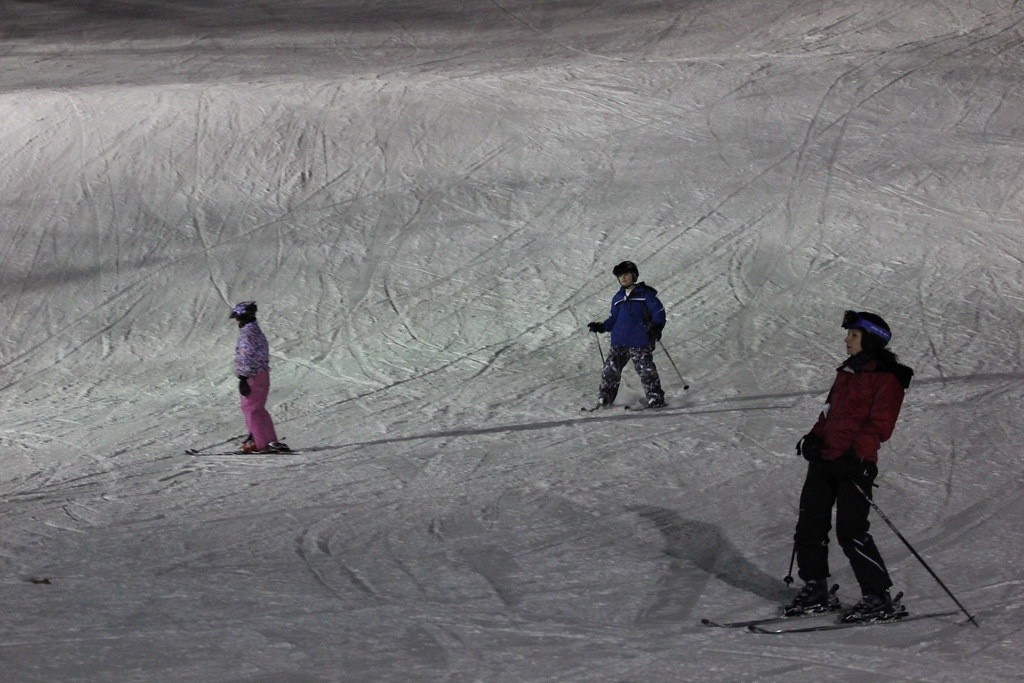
853 589 892 613
792 580 829 607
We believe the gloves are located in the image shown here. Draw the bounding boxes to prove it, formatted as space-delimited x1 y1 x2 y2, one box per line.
588 322 606 333
238 375 250 396
802 432 823 463
845 459 878 499
648 323 665 343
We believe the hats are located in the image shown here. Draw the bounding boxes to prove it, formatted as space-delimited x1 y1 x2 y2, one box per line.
842 312 891 353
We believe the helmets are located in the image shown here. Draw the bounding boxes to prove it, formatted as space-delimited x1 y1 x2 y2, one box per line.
230 300 256 319
613 261 639 284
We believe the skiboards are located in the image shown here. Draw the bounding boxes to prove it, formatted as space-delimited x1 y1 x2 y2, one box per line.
699 606 960 636
184 433 303 455
580 402 669 412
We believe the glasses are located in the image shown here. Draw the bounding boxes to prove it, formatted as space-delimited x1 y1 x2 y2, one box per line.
843 310 858 324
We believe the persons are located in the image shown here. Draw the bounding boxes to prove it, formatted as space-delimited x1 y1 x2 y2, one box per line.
588 261 667 409
782 310 915 614
229 300 276 453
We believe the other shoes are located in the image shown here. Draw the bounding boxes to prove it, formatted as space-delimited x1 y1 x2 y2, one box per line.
647 395 665 408
252 446 270 453
595 397 610 408
